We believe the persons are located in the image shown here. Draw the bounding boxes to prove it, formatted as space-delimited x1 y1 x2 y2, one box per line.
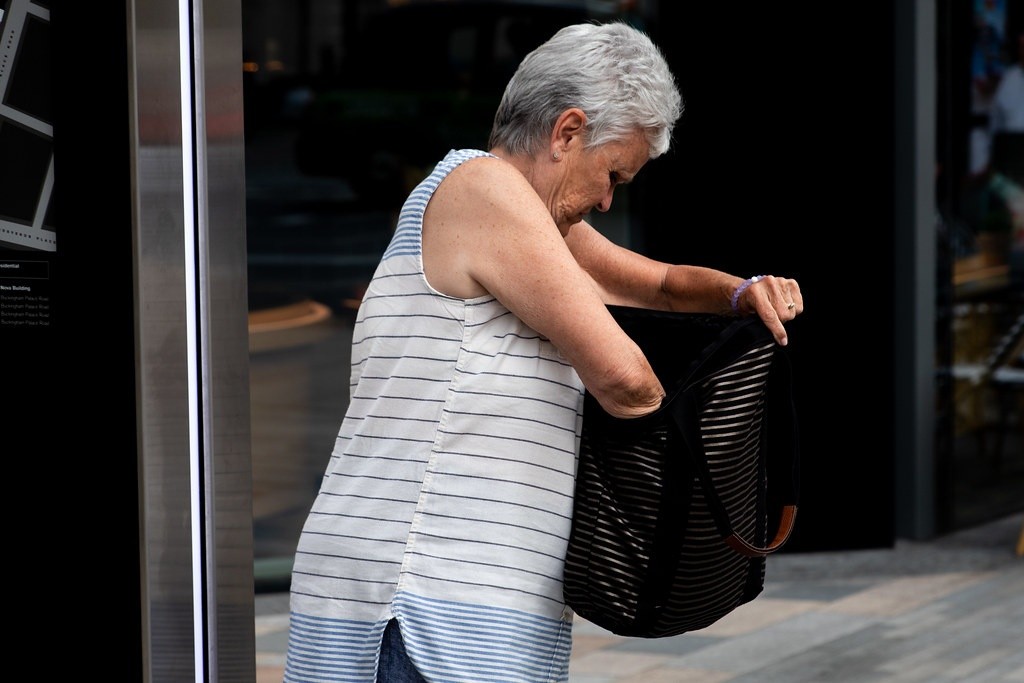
287 23 804 683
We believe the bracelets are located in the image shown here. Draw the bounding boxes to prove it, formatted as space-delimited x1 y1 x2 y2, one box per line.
730 275 766 310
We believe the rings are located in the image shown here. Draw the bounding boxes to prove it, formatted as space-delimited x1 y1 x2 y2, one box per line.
788 302 796 310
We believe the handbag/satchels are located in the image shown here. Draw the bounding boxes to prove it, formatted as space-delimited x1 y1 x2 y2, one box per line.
559 293 804 639
